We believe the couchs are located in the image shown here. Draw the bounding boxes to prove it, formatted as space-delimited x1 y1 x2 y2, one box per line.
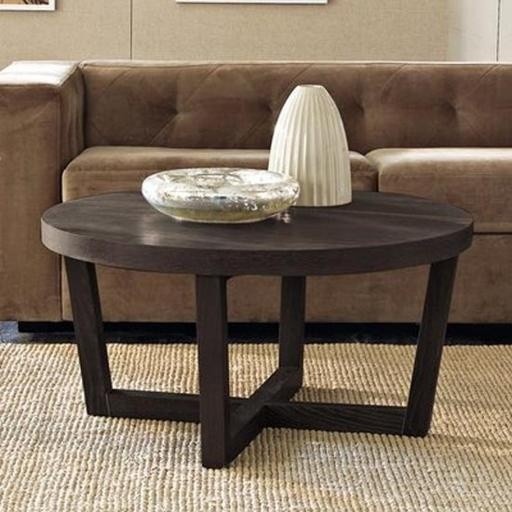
0 61 512 333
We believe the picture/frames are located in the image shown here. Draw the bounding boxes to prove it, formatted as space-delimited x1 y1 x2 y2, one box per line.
0 0 55 11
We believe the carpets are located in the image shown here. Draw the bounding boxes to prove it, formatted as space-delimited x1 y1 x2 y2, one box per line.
0 343 511 512
